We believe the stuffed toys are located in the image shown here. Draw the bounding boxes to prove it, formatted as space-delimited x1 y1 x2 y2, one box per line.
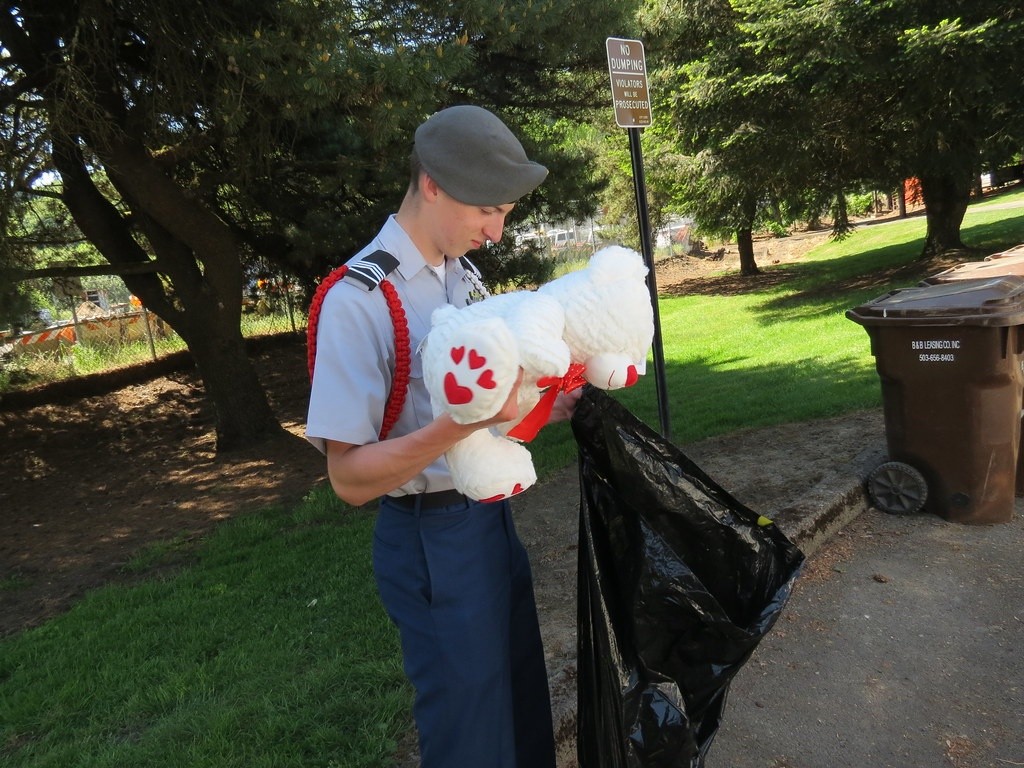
415 243 657 504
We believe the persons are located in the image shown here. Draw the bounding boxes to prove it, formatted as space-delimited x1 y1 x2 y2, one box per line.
305 105 553 768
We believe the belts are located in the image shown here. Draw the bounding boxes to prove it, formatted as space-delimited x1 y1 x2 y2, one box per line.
385 487 465 511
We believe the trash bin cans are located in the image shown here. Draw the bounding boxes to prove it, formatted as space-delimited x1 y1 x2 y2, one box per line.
847 243 1023 525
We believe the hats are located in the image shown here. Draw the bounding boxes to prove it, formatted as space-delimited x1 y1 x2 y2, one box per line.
413 106 549 207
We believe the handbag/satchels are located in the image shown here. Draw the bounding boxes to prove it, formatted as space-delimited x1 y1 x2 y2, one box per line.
577 386 805 767
